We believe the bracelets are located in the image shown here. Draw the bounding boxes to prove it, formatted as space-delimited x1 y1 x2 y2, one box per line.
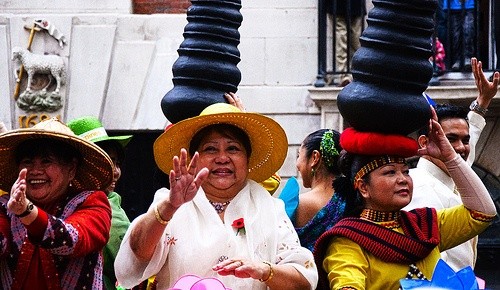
154 205 172 224
259 261 273 283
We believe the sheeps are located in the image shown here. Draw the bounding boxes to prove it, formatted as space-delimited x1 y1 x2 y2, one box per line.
10 46 66 93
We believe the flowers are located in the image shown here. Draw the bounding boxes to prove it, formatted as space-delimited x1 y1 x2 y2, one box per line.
231 218 246 236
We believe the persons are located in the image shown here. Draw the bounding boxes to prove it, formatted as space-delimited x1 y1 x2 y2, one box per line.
313 120 497 290
402 57 500 280
62 115 134 290
114 103 319 290
0 115 117 290
223 91 281 195
278 128 347 252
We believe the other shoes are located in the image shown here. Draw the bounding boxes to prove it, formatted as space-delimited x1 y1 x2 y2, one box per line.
452 59 462 71
338 75 351 86
463 57 472 71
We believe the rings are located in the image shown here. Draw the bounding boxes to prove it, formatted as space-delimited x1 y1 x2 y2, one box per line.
175 177 182 181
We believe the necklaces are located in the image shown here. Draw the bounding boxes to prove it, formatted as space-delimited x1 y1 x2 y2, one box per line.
206 200 234 213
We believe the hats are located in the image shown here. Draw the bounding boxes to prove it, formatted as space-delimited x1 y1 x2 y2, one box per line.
153 103 288 183
66 116 133 149
0 117 114 192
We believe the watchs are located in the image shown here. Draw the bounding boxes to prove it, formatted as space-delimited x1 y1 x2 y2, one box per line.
468 101 488 114
15 201 34 218
